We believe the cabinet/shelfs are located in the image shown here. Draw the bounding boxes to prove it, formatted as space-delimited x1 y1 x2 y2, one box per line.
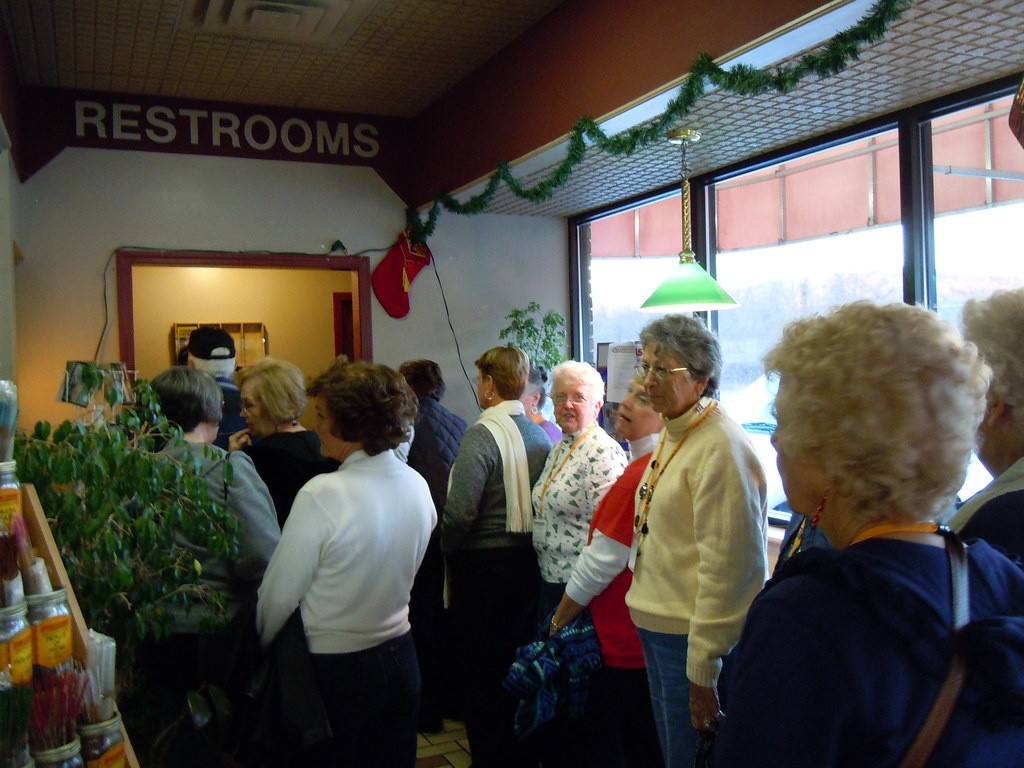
172 322 267 364
0 481 139 768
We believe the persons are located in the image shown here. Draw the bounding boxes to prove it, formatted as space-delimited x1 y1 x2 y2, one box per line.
181 326 248 450
943 285 1024 566
624 317 769 767
531 360 630 605
229 361 337 531
439 346 552 684
716 302 1024 767
151 367 281 768
522 366 562 446
398 359 469 642
255 363 440 768
550 374 664 768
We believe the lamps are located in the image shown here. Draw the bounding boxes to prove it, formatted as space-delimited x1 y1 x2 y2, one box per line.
640 128 740 310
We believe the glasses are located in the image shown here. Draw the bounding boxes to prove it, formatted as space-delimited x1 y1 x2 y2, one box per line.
634 364 690 381
552 393 589 406
473 377 484 385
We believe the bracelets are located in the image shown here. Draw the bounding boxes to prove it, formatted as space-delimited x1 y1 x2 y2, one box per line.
550 616 558 632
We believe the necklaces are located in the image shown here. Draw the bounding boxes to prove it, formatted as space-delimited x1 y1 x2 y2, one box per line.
635 461 659 558
849 517 887 542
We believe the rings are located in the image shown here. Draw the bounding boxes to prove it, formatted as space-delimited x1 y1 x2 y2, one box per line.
704 720 711 726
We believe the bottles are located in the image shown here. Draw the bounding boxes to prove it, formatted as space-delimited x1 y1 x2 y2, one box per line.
20 709 127 768
0 458 24 537
0 602 32 688
26 584 73 672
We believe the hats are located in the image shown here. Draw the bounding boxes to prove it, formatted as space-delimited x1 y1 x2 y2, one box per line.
188 327 236 360
